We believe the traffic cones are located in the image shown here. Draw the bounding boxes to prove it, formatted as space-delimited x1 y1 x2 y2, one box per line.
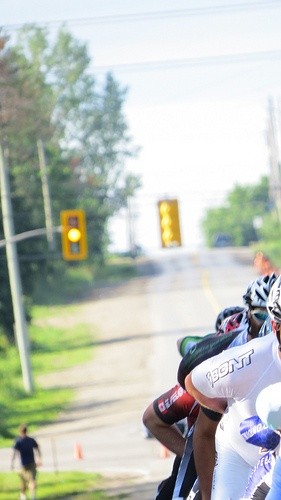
75 445 83 461
162 443 170 459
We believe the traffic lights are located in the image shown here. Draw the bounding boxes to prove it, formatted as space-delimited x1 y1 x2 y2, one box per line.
60 207 88 261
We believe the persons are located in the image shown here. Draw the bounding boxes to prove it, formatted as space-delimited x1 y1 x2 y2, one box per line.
10 425 43 500
142 272 281 500
